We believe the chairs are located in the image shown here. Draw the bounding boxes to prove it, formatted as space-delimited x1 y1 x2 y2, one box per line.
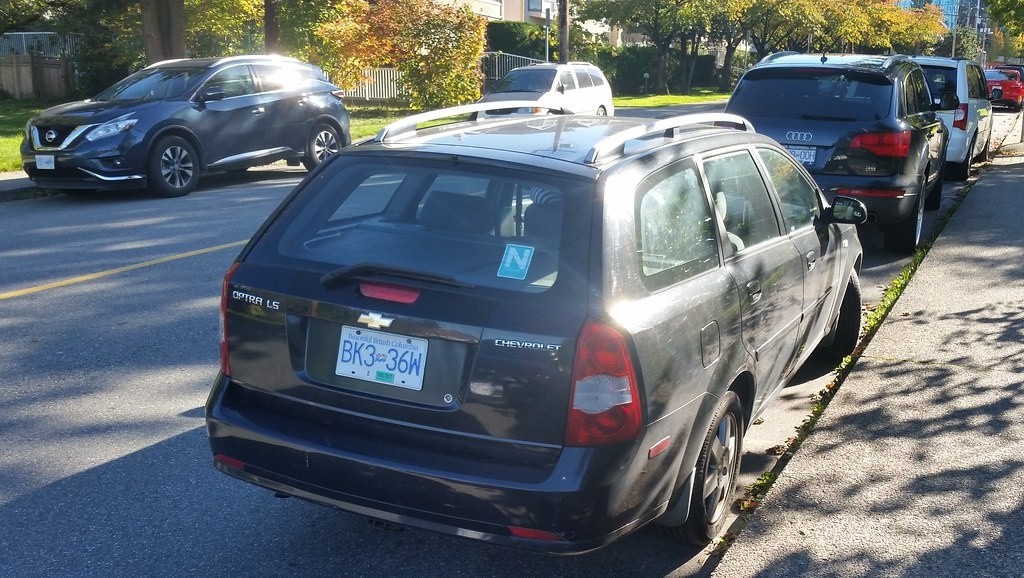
523 204 558 237
714 190 745 251
420 191 499 233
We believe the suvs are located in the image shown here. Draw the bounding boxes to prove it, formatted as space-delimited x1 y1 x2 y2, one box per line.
204 99 865 561
903 56 1004 181
19 54 354 199
469 59 614 121
712 50 962 255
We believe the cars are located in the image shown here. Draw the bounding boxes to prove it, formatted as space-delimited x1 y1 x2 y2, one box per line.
994 64 1024 84
982 68 1024 111
